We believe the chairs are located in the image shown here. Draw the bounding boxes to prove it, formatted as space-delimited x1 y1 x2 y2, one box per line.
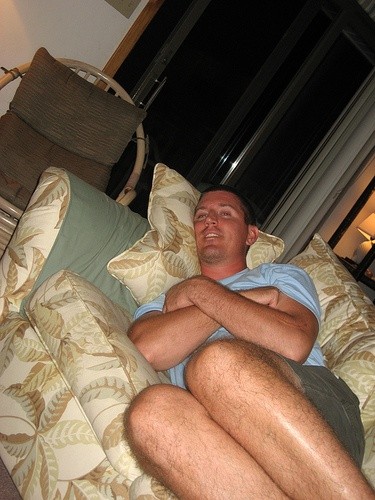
0 58 145 258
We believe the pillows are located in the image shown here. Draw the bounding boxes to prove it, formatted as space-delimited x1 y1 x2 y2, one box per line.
146 160 283 281
0 47 147 212
108 230 184 307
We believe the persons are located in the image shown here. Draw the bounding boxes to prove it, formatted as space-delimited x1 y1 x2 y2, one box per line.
124 187 375 500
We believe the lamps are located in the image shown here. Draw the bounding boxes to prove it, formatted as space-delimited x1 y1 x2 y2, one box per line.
343 212 375 269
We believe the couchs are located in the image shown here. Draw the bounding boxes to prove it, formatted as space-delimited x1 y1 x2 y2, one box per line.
0 167 375 500
